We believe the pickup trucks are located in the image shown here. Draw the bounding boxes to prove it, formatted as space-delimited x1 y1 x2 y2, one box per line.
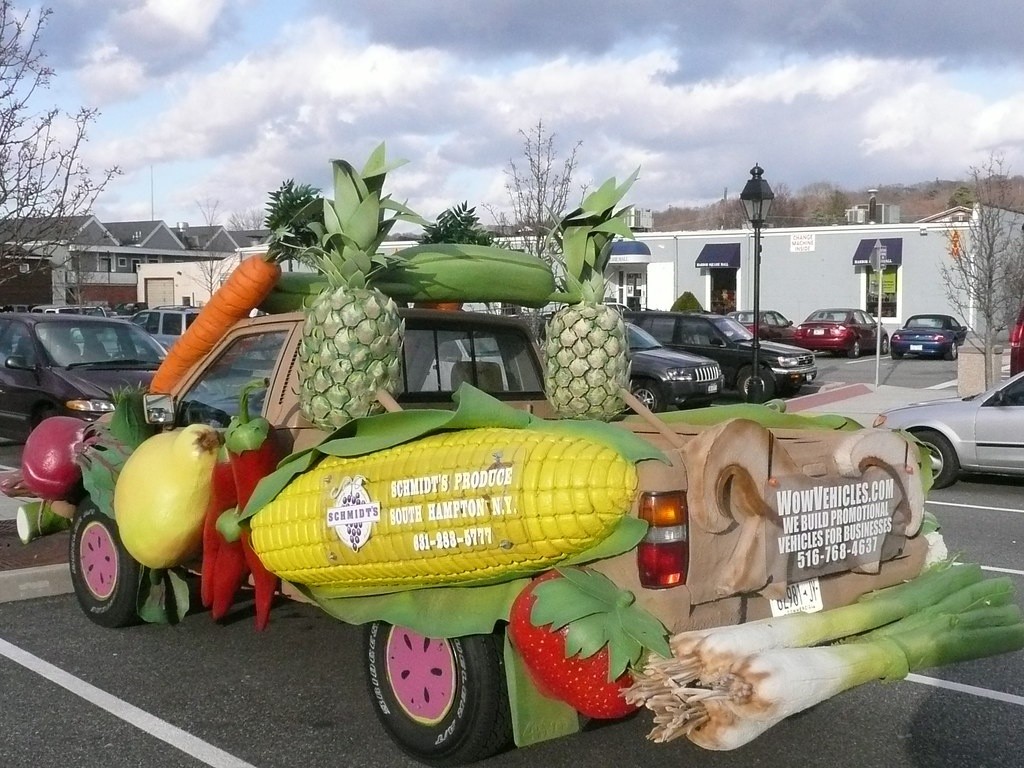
70 309 944 764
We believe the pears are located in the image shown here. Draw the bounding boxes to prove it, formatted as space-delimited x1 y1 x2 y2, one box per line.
114 422 224 570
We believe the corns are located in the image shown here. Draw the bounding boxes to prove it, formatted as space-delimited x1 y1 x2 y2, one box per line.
231 381 668 607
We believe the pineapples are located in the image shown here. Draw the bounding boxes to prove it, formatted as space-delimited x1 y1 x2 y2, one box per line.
546 163 643 422
300 136 430 433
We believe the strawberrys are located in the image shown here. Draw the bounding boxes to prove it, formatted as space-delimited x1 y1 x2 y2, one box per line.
508 566 674 719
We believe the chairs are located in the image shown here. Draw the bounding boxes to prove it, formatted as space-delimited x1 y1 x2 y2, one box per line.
694 325 712 344
826 315 834 320
18 336 40 355
451 361 503 392
84 342 107 356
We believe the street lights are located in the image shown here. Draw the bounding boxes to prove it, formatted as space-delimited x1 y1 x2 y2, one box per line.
739 162 775 405
873 371 1024 490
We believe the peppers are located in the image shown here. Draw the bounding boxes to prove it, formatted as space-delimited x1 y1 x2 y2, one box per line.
201 377 284 632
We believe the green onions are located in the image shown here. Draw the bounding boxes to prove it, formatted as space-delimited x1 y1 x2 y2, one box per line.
622 556 1024 749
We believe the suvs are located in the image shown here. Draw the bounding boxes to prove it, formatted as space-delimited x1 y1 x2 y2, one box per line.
623 307 817 403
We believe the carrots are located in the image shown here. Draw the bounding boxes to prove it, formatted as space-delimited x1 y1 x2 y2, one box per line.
146 252 281 394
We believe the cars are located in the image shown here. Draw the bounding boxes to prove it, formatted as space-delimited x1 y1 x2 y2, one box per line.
890 314 968 361
795 308 889 359
508 302 725 415
727 310 797 345
0 305 242 443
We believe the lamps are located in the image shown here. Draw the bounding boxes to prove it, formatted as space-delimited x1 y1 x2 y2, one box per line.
920 227 928 235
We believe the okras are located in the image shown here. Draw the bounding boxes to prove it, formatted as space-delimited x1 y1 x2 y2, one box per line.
252 241 558 314
